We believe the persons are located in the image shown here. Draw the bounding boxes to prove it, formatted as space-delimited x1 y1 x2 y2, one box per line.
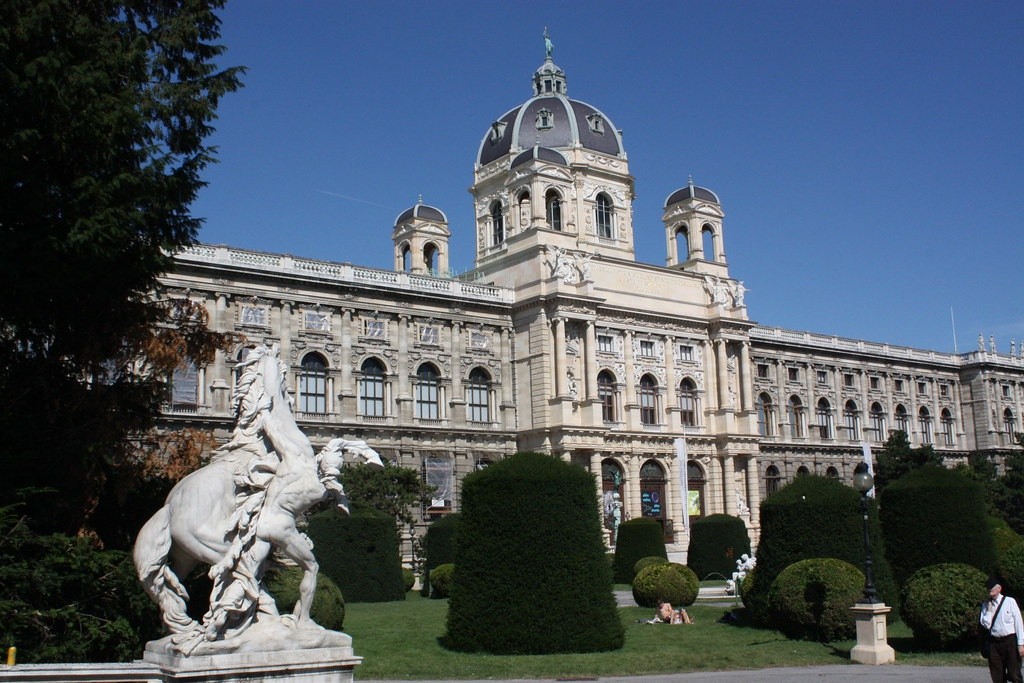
608 492 624 546
1019 339 1024 357
644 599 695 624
988 334 997 354
979 575 1024 683
977 333 986 351
1010 338 1017 357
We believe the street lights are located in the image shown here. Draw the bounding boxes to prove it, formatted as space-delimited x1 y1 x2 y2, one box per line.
409 522 421 591
851 460 894 665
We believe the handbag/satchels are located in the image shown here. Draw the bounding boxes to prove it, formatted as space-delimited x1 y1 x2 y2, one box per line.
977 619 992 658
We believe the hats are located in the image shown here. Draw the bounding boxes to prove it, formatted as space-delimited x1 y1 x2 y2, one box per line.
983 577 1001 588
